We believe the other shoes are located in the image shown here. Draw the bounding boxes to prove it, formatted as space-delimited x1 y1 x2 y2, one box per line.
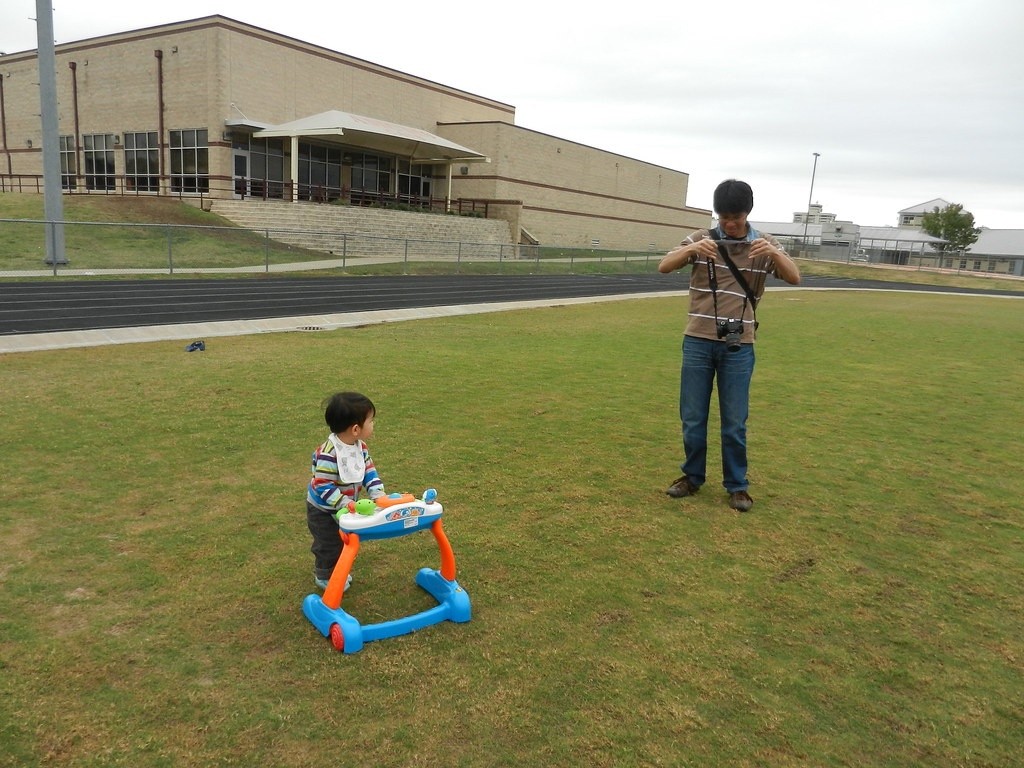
314 573 352 592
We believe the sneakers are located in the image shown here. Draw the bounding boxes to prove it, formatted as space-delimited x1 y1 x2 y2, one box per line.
728 491 753 511
666 475 700 497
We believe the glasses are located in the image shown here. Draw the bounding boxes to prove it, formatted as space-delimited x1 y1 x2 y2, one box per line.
716 214 746 224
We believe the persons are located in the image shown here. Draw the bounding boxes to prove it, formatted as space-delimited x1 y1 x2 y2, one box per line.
305 392 389 593
658 180 800 512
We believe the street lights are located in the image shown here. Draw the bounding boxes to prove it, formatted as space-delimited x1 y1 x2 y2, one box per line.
798 153 820 258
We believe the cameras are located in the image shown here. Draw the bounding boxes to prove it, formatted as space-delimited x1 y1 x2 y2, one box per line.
717 317 744 353
